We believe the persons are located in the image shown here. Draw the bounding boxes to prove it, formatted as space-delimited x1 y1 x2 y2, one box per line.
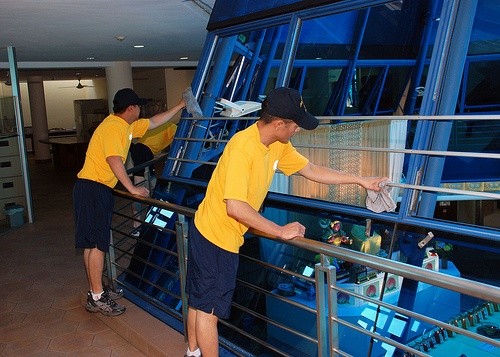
73 87 188 317
185 87 392 357
315 218 353 273
131 122 181 236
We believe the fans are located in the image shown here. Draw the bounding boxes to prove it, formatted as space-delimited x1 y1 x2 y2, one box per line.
57 73 96 90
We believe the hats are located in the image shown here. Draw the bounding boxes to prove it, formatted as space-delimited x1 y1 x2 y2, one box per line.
113 88 149 106
261 87 319 130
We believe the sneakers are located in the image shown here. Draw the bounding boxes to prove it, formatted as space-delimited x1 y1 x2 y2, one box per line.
184 352 202 357
85 285 126 316
130 227 141 237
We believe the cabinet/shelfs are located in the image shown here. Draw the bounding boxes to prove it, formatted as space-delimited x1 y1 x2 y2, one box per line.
0 131 28 224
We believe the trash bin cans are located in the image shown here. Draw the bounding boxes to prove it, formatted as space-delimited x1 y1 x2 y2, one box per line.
5 204 24 228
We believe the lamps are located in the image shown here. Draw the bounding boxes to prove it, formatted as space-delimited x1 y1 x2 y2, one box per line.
216 93 264 118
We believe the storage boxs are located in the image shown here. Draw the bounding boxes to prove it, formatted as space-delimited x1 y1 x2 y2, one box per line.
292 276 316 302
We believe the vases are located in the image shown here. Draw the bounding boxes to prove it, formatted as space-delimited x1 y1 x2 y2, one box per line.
442 257 447 269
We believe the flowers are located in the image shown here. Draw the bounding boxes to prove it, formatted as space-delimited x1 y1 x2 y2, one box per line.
435 240 455 258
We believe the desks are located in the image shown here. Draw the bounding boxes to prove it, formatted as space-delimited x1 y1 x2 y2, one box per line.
38 134 88 174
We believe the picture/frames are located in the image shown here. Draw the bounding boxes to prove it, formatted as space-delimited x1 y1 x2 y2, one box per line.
325 283 360 308
380 274 398 293
423 258 438 272
359 278 381 304
